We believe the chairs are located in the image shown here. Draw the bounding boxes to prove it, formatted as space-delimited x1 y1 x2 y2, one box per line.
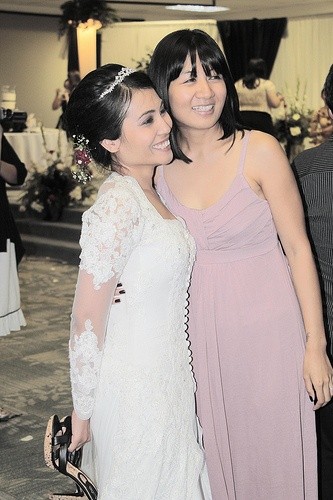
238 111 275 135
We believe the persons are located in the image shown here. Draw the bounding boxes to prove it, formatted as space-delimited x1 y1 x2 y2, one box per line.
230 55 286 142
52 79 73 141
1 124 28 426
62 62 212 500
289 64 333 500
146 28 333 500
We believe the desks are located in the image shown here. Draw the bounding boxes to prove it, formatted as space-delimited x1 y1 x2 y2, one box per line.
4 127 69 187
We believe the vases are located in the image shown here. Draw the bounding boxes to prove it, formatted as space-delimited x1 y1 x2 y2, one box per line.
284 144 306 164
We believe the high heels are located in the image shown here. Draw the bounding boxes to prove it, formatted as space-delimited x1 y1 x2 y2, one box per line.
43 414 98 500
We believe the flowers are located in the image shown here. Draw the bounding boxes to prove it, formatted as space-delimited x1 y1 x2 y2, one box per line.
67 133 96 184
278 75 315 145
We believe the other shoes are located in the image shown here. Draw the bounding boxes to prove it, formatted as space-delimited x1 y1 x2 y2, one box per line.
0 407 9 418
